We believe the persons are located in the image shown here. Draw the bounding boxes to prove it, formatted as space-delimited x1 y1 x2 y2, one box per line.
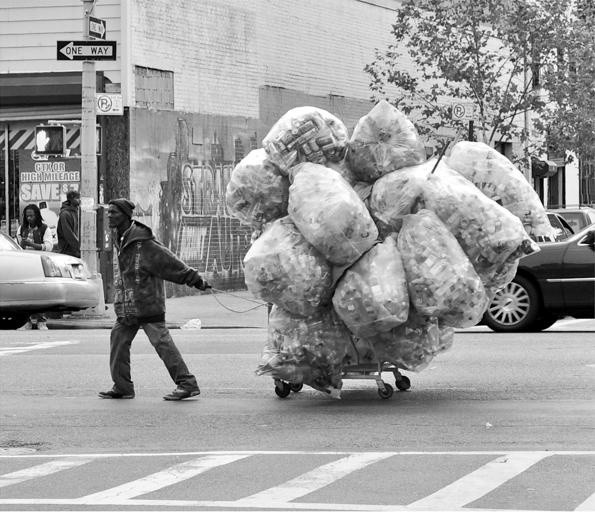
15 204 53 252
56 191 81 256
98 198 210 398
37 202 58 243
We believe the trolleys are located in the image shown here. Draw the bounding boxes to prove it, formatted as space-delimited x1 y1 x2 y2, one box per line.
257 314 413 400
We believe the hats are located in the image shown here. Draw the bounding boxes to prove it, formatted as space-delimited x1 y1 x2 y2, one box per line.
108 197 136 219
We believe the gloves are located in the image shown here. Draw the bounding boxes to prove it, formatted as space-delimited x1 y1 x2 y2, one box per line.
195 279 212 291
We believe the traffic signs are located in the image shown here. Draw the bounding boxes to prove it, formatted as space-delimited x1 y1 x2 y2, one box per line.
57 40 117 62
88 17 106 40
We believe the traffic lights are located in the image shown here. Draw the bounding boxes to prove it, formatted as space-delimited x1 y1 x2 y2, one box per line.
35 123 67 156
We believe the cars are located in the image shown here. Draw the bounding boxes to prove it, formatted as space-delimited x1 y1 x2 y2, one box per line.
1 231 102 330
478 223 595 333
519 206 594 243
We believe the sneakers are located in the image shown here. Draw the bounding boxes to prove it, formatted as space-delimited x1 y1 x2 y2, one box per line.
98 389 135 399
163 386 200 400
